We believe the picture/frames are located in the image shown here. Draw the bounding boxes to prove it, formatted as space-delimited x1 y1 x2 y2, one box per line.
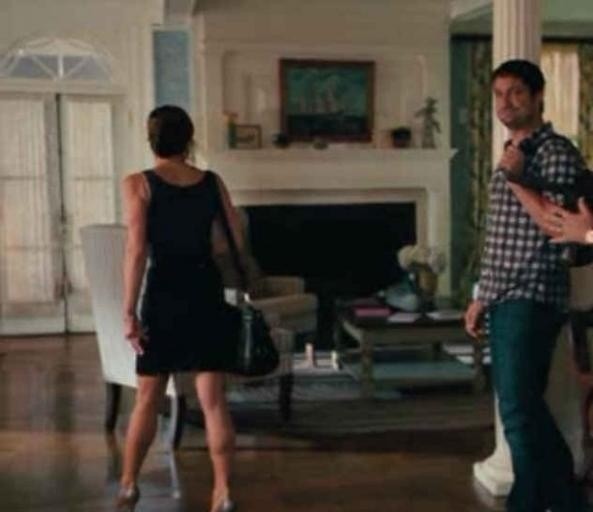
234 125 262 151
279 57 375 144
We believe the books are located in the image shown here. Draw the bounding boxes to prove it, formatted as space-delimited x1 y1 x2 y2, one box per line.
352 303 469 324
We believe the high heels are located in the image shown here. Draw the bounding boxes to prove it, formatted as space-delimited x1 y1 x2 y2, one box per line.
213 499 236 512
115 485 139 511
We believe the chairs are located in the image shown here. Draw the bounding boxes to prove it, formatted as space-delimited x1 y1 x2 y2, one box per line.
79 225 293 451
211 209 319 368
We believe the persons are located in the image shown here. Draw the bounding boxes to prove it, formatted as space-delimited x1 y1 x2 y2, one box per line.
465 58 583 512
115 106 243 511
545 196 593 246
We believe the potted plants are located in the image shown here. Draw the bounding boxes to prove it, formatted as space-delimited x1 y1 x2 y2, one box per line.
391 126 412 148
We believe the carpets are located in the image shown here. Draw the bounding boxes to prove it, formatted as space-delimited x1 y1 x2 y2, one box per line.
186 349 494 435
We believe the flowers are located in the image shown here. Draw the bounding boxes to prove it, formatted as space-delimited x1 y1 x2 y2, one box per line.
396 244 449 274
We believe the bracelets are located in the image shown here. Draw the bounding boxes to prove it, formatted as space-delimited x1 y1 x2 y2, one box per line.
584 229 593 244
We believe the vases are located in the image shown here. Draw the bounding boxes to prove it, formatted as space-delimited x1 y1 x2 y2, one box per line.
406 260 439 311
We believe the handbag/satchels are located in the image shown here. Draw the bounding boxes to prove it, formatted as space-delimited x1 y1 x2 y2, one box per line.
560 166 592 269
233 301 280 378
